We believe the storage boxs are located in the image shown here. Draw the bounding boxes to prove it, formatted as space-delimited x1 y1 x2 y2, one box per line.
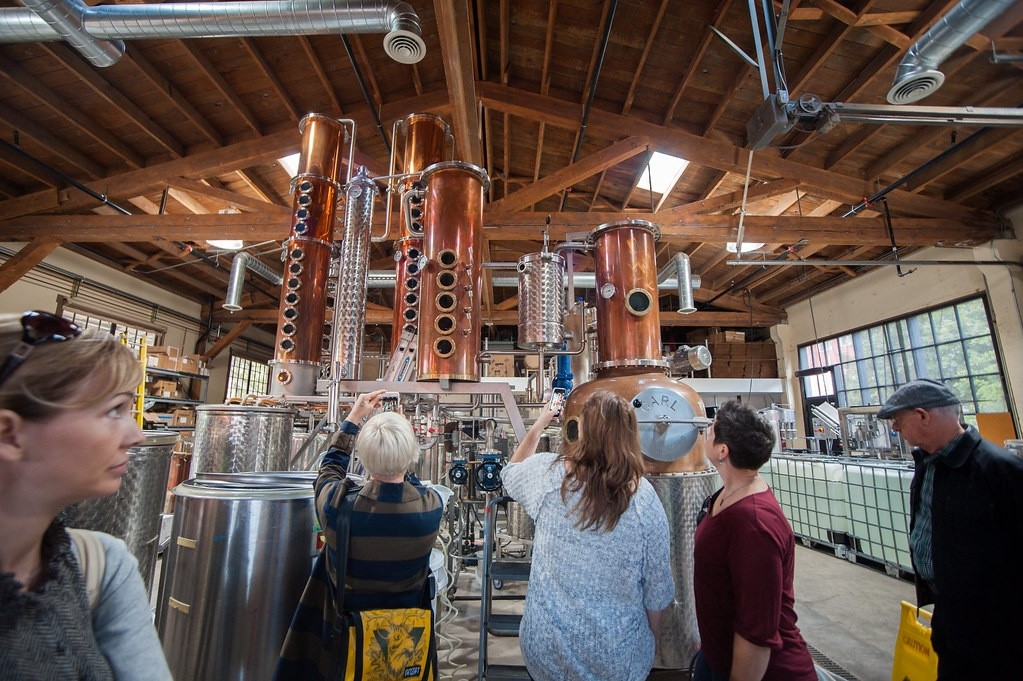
148 346 179 371
145 382 161 397
156 413 173 427
191 378 207 401
173 410 193 427
142 413 161 429
148 353 168 371
151 379 176 398
363 354 379 380
487 353 515 378
180 355 200 373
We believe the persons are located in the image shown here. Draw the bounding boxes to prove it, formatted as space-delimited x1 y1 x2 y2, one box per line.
1 308 172 681
691 401 821 679
271 388 445 680
501 384 674 676
877 373 1022 680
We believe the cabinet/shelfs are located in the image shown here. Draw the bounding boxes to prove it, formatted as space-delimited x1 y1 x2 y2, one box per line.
119 333 210 433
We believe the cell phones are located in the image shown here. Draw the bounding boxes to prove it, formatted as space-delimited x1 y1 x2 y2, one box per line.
549 387 566 419
378 392 400 415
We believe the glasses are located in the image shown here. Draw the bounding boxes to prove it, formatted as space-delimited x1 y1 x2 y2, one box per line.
0 309 82 384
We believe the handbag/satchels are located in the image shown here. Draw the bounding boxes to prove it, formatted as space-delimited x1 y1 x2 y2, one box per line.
328 606 438 681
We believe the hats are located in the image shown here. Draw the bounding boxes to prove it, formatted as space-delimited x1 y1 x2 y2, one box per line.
876 378 960 420
